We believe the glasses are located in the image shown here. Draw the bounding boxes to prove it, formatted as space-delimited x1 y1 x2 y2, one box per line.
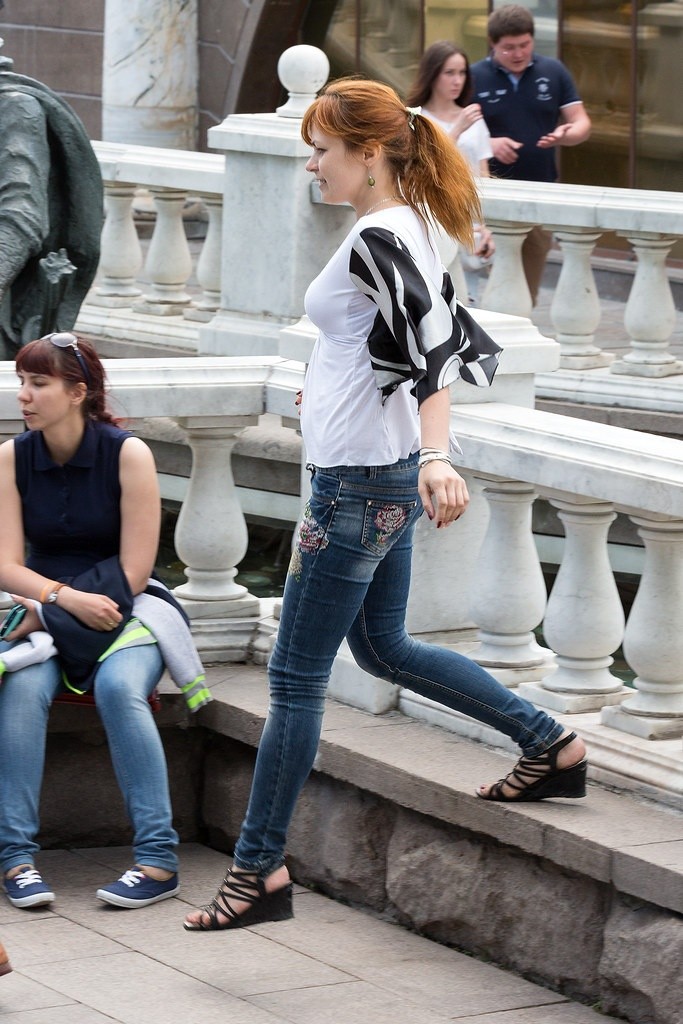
40 331 95 391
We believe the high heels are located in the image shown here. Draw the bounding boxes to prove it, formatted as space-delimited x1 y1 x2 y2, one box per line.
183 860 293 931
476 731 587 802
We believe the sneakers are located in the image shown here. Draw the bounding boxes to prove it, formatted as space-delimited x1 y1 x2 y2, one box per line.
97 864 180 909
1 866 54 907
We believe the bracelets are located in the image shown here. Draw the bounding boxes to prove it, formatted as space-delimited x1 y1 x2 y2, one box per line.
418 447 451 468
48 584 66 604
40 581 57 603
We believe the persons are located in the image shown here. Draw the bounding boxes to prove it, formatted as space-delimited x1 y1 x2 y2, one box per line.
469 4 593 305
0 331 214 910
0 28 106 358
183 76 588 932
404 42 494 300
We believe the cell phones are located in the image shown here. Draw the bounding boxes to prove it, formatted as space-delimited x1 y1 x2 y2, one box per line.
0 604 24 637
